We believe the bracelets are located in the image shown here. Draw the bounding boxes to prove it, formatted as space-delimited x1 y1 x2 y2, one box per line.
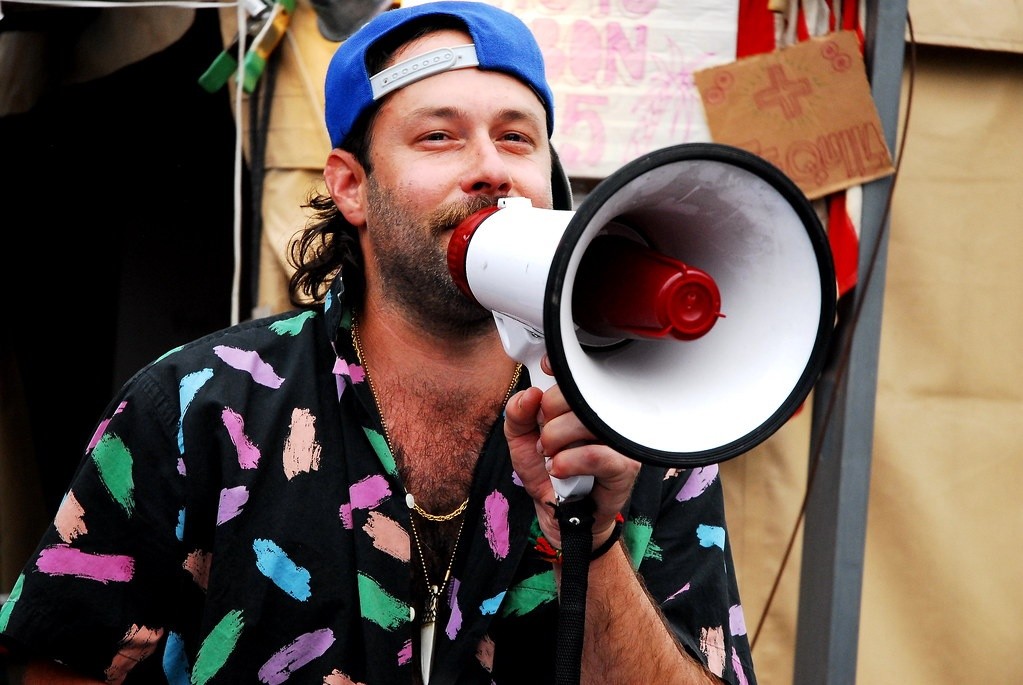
528 513 625 564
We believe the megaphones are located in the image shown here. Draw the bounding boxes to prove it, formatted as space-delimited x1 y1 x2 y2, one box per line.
448 140 837 503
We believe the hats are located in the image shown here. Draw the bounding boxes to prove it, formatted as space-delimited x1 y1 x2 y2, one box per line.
323 2 576 219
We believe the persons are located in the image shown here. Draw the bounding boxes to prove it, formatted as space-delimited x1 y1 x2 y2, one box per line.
0 2 756 685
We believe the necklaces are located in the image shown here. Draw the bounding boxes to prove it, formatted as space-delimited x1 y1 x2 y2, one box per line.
350 315 524 685
